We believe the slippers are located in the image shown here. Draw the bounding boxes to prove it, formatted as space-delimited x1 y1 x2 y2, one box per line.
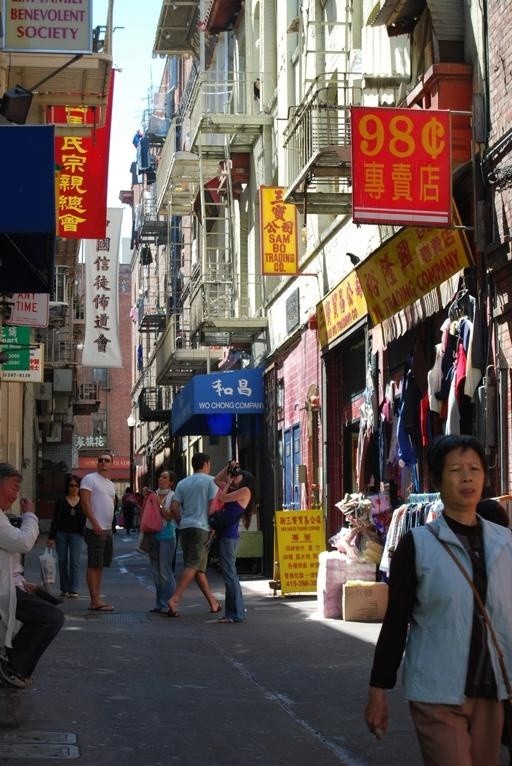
88 604 113 611
219 617 235 623
158 610 180 618
211 602 222 613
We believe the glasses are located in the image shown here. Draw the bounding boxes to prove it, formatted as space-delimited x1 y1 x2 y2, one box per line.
69 484 79 487
98 458 110 462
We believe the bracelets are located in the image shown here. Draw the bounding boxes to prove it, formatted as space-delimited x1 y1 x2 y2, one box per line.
159 504 164 510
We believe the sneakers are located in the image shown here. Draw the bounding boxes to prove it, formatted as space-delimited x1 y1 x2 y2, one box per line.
60 591 68 596
0 663 27 687
70 591 79 597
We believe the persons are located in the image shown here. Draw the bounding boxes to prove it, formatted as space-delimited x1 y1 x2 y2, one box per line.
213 461 255 623
143 471 179 614
78 452 115 612
121 487 138 534
112 489 118 533
363 435 512 766
46 476 83 599
167 453 221 614
474 499 509 530
1 462 66 688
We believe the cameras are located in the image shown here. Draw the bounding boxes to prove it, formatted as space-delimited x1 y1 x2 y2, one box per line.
227 463 238 478
